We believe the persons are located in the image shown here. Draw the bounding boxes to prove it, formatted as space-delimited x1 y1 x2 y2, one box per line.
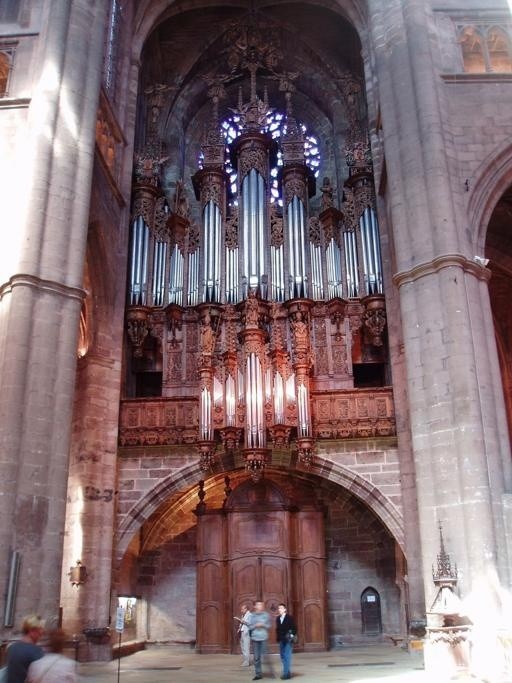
274 603 297 680
233 604 251 667
0 613 82 683
247 600 275 681
